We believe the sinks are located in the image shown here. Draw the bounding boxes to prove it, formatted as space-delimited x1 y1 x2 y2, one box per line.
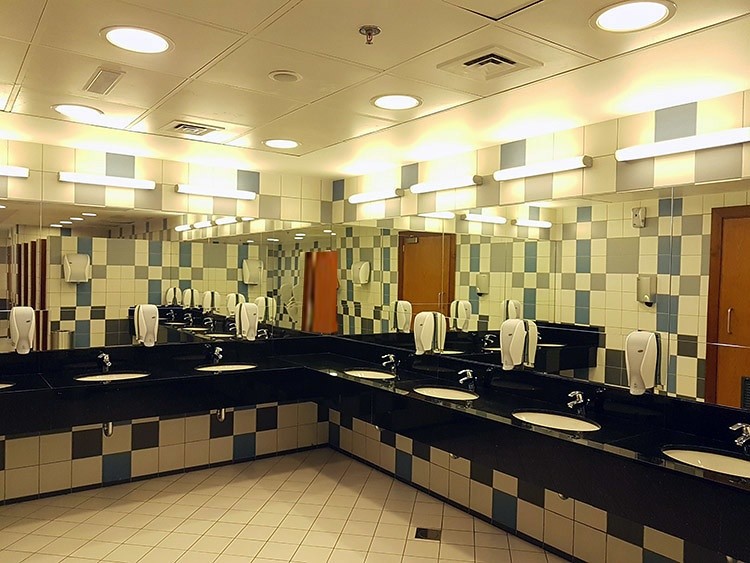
164 321 236 338
482 347 501 351
536 343 566 347
414 386 479 401
344 368 396 381
433 349 463 355
510 409 601 431
662 443 750 479
195 363 258 372
75 371 151 381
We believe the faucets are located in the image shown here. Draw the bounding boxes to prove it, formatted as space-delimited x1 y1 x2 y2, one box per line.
537 332 542 339
458 369 478 391
98 353 112 373
566 389 591 413
481 333 496 346
165 309 270 339
380 354 400 372
730 423 749 447
211 346 224 363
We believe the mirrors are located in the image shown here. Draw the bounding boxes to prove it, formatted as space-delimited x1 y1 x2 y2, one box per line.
329 176 749 410
0 198 329 353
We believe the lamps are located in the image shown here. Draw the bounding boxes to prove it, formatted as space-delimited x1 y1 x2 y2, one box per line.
174 184 256 200
410 175 483 194
614 127 749 162
57 171 156 190
348 188 404 204
418 211 551 229
492 155 593 182
173 216 257 231
0 165 30 178
